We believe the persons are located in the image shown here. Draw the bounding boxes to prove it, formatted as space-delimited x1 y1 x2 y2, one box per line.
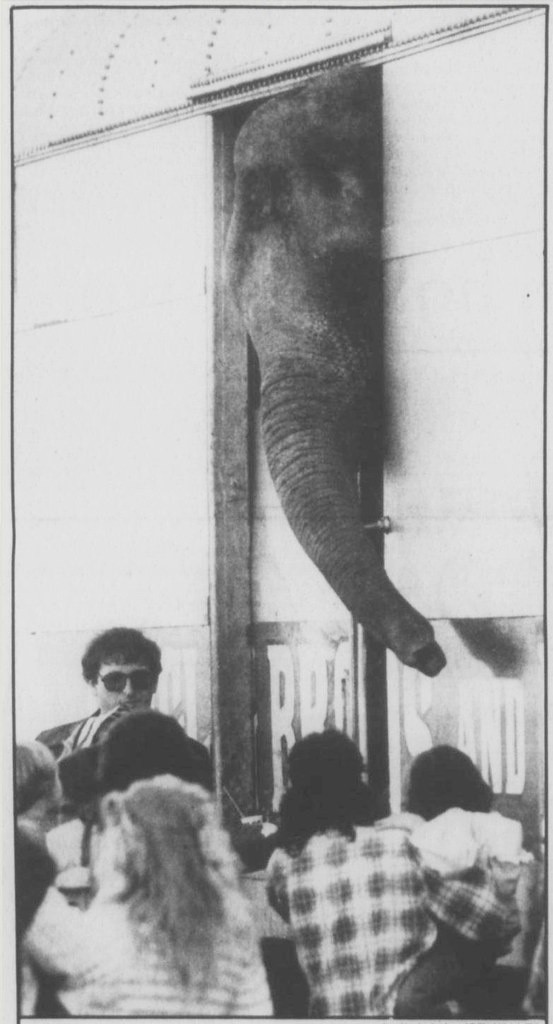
378 743 540 1021
34 627 215 792
14 711 545 1019
55 776 274 1018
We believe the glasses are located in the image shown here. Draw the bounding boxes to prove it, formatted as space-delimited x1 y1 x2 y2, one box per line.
99 669 156 691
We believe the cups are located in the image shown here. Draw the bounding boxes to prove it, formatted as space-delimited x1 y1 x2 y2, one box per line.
241 815 263 824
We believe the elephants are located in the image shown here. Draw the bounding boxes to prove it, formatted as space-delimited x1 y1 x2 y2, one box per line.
222 61 447 681
